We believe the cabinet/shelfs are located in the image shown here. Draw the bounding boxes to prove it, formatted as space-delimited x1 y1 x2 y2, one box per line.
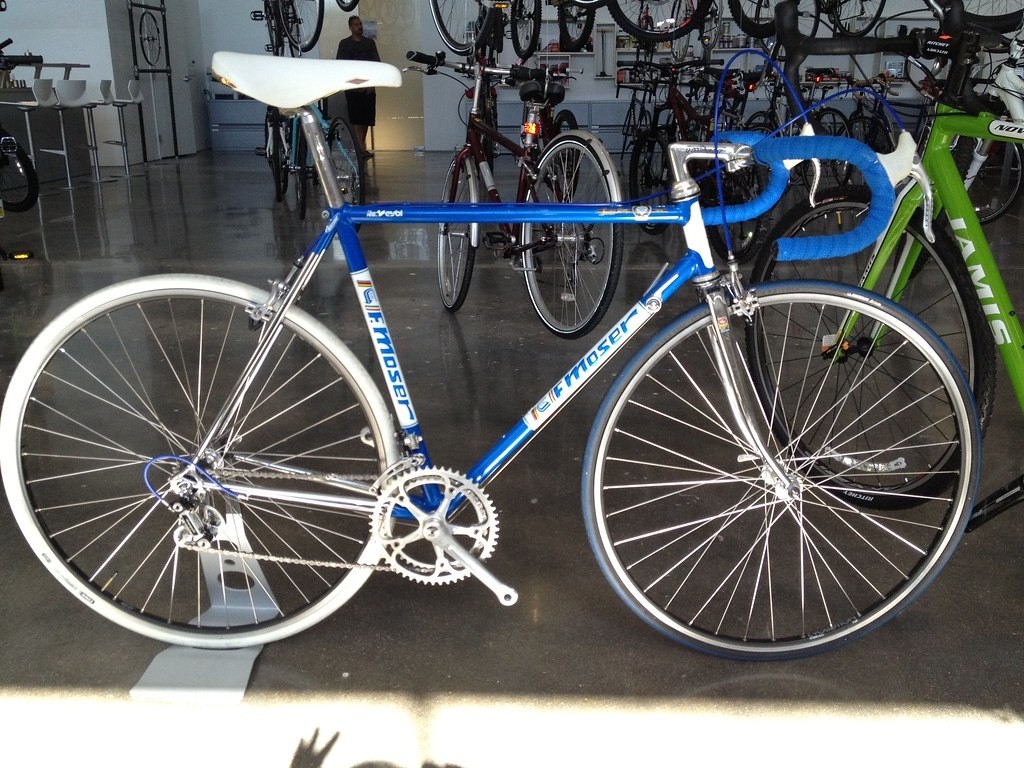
204 69 269 152
465 0 938 155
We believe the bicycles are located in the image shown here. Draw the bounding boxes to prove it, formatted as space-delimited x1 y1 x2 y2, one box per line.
0 38 43 213
0 0 1024 662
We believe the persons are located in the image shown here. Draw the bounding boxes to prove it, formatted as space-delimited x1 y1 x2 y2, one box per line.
336 15 380 161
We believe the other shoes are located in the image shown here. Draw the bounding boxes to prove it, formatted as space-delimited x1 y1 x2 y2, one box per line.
362 150 374 160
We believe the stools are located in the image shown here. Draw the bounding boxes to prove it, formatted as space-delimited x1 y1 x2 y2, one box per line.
0 79 57 197
41 80 91 190
81 80 117 184
99 79 143 179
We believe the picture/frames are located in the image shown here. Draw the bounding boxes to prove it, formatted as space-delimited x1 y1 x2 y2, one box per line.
885 61 905 77
